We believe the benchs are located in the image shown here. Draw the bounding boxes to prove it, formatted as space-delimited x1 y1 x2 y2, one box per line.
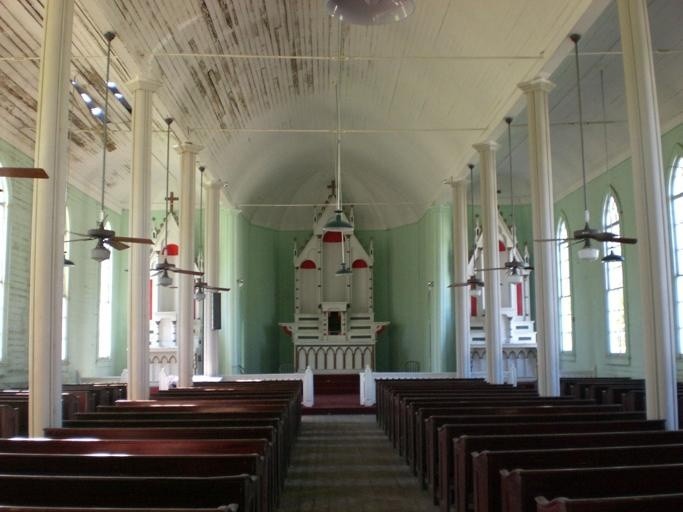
0 382 127 439
558 376 683 428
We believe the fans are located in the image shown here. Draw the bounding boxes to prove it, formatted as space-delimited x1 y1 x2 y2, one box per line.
473 117 538 271
59 29 156 251
145 116 204 276
446 163 489 285
534 30 638 246
168 163 230 291
0 166 49 179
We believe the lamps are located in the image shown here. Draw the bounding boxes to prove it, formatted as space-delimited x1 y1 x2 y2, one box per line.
90 247 111 263
63 251 76 266
335 233 356 276
599 71 626 264
157 276 173 287
576 247 600 260
506 274 523 283
193 292 207 302
469 289 482 296
0 189 6 206
308 0 422 29
323 82 356 232
